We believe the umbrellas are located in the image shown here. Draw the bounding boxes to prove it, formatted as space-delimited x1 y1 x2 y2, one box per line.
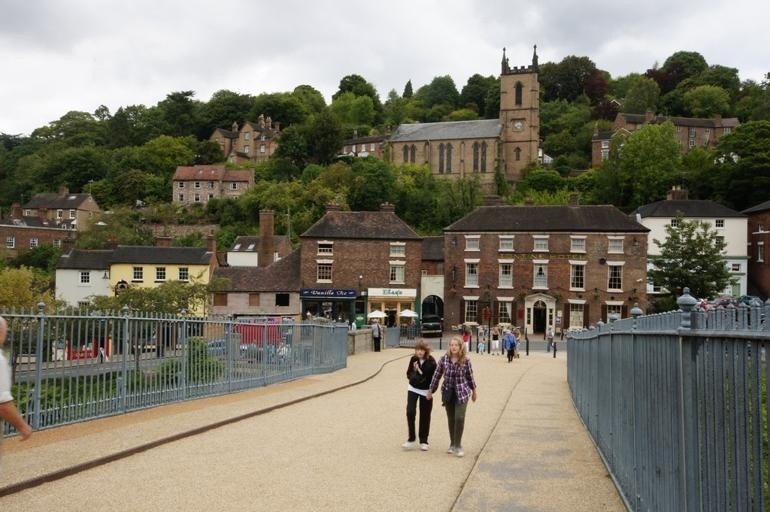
398 309 418 324
368 310 388 323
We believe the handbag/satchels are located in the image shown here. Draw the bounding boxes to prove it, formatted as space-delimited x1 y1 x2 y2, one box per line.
441 387 456 405
509 343 516 348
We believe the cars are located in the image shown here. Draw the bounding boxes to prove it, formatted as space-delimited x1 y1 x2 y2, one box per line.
207 339 226 355
132 329 157 352
420 315 442 337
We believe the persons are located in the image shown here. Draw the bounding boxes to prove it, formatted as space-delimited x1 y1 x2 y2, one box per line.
0 315 32 442
351 319 356 330
460 327 520 363
426 337 476 457
400 338 437 451
546 326 556 353
372 319 381 352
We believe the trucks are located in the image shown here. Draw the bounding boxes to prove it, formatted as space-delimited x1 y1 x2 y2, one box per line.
237 317 294 347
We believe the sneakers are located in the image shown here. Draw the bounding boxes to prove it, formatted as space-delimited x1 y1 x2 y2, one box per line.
401 440 429 451
447 446 464 457
